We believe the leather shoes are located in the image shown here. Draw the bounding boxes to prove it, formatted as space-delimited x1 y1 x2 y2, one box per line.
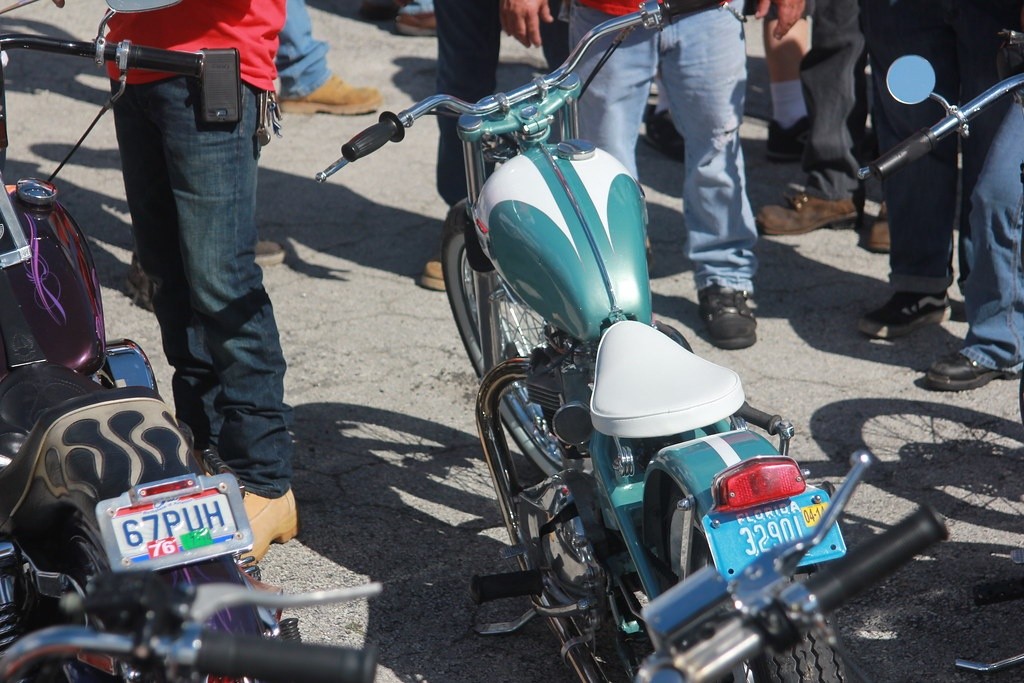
927 353 1023 390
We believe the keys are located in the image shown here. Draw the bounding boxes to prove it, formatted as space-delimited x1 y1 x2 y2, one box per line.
267 89 281 127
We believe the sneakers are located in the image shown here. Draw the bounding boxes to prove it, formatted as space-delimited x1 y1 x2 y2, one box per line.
756 191 858 235
858 291 952 337
699 284 757 349
279 76 378 115
868 204 891 250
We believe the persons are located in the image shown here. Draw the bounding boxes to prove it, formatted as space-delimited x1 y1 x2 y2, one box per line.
55 0 303 569
274 0 1024 391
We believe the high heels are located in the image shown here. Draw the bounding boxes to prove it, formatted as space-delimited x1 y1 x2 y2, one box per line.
239 487 298 568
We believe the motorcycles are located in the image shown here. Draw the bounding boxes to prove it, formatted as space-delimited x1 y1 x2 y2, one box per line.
1 0 304 681
317 0 847 682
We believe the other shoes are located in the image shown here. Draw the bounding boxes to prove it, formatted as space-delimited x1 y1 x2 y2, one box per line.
643 104 685 162
254 239 286 265
125 252 155 311
420 250 447 290
766 116 811 161
396 4 438 35
